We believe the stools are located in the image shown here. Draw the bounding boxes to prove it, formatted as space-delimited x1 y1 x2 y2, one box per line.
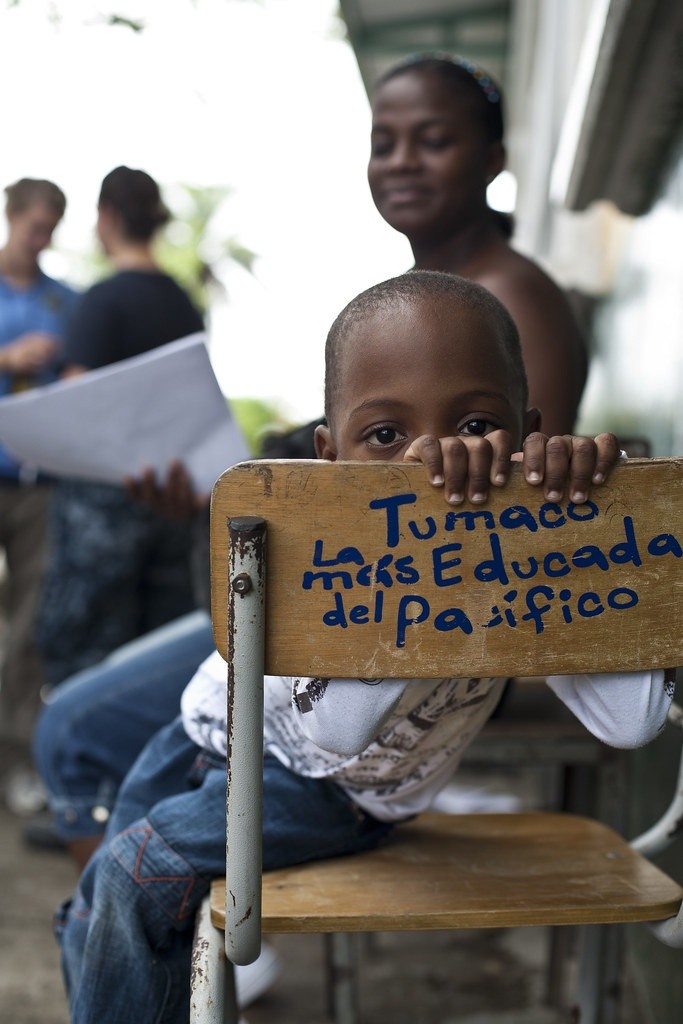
307 716 608 1022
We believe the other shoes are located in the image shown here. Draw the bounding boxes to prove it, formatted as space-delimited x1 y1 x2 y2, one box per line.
7 775 46 816
20 829 75 855
231 946 277 1007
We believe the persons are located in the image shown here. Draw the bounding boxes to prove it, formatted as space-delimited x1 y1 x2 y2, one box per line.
54 272 629 1024
32 57 587 1005
0 179 77 820
25 164 204 852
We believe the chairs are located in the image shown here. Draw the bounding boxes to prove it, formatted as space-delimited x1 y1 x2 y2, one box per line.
189 459 683 1024
